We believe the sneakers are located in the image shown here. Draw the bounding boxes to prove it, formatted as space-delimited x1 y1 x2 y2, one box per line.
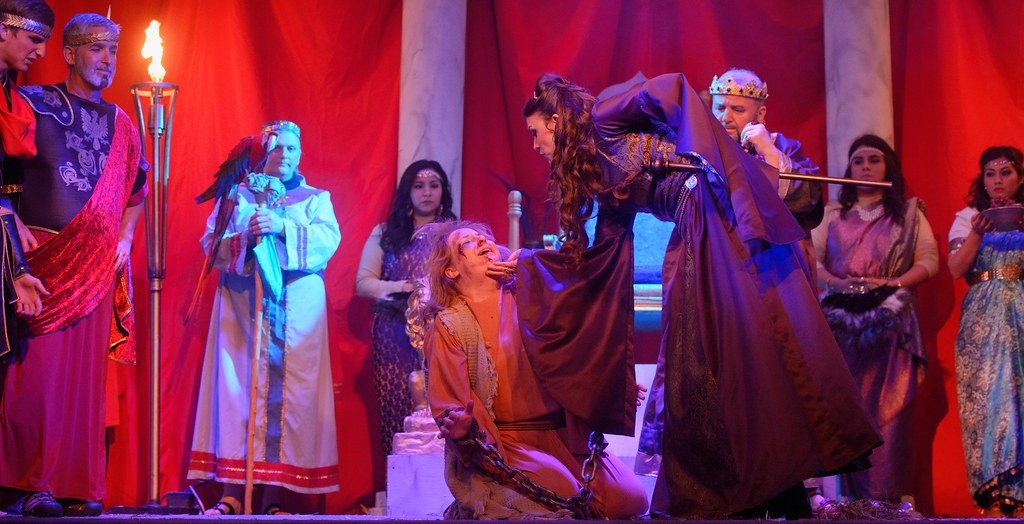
61 498 103 517
24 491 62 516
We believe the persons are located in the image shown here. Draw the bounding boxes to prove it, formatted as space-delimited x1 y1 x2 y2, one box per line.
698 68 830 230
0 0 55 364
188 120 341 517
808 133 939 507
946 145 1024 519
357 157 466 458
485 71 884 523
411 215 653 521
1 14 141 517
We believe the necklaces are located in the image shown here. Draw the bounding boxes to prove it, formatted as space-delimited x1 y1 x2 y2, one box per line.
854 198 883 221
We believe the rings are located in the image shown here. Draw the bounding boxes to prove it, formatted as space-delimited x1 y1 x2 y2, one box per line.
744 136 749 139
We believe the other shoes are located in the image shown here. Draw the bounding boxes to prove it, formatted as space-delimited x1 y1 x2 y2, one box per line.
986 503 1005 517
1011 504 1024 518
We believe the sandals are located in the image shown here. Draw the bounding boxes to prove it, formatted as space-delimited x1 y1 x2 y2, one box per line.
263 504 293 516
204 500 242 515
808 489 842 520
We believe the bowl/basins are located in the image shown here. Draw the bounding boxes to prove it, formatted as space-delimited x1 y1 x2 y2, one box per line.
981 205 1023 232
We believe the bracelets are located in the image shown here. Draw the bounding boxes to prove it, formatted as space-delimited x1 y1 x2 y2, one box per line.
895 278 901 288
825 275 832 290
